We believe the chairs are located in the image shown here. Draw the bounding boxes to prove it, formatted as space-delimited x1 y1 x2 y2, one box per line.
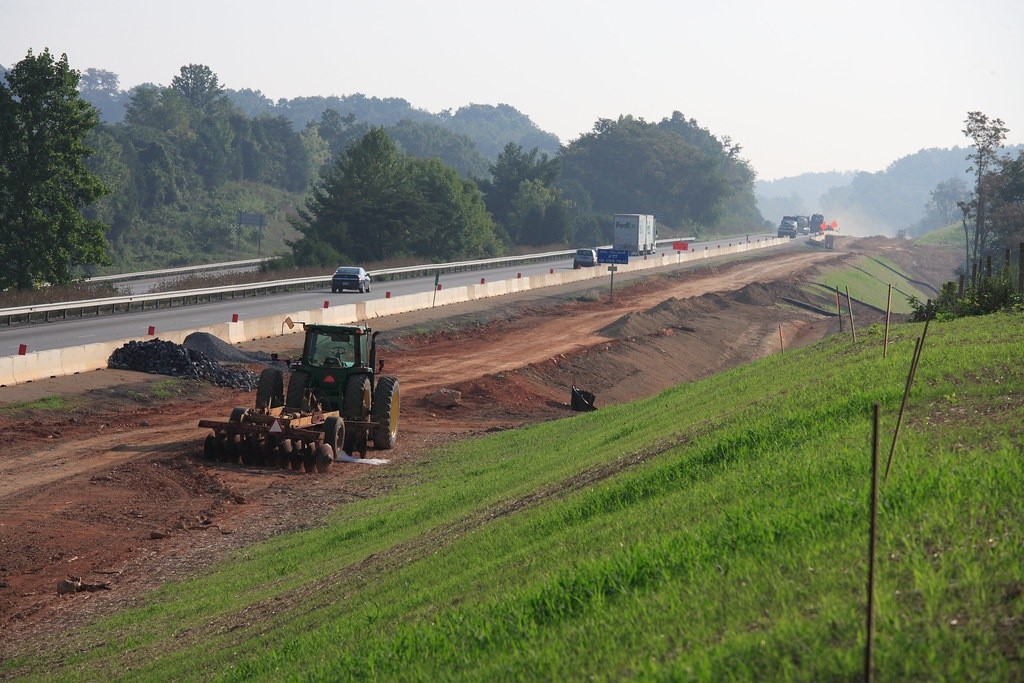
320 356 343 367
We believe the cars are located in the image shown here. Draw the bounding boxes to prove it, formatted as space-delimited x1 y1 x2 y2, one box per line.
573 248 601 268
330 267 370 293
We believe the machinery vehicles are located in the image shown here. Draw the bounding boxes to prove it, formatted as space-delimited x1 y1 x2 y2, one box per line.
198 322 401 475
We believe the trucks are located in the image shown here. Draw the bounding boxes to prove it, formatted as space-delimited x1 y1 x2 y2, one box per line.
778 213 824 240
614 213 659 256
